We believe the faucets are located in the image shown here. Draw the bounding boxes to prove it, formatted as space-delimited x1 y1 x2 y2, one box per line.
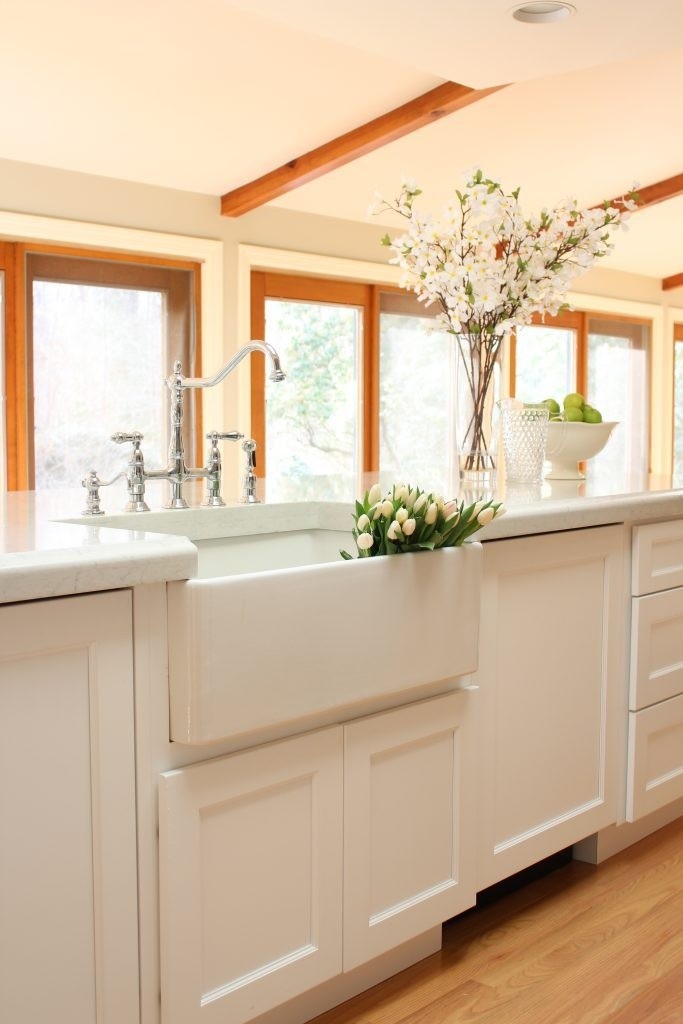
240 438 262 504
111 338 286 512
80 468 131 516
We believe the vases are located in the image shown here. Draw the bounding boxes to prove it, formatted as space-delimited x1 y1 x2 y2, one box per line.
449 333 502 481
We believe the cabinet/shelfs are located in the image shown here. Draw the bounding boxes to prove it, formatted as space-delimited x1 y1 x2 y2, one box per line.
2 530 197 1022
470 498 682 896
157 545 474 1023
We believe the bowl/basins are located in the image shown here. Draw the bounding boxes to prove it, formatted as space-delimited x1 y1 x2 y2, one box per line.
541 420 621 480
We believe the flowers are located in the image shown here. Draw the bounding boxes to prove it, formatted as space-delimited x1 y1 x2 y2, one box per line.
343 482 504 559
384 166 644 473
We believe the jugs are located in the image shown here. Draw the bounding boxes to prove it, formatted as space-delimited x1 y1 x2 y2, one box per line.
494 398 568 487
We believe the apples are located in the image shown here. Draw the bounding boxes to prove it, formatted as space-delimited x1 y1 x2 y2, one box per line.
543 394 603 423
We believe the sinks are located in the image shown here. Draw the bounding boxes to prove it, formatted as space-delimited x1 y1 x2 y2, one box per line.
166 528 485 747
